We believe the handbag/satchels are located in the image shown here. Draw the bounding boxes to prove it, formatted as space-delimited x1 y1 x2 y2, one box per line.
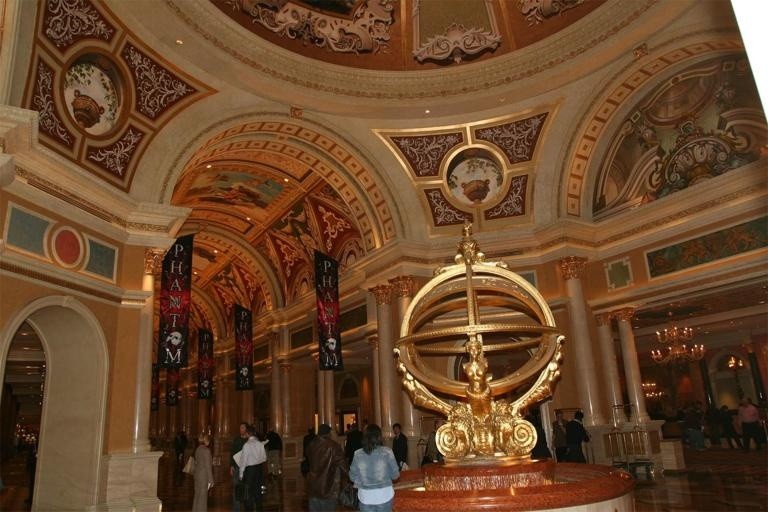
182 456 196 474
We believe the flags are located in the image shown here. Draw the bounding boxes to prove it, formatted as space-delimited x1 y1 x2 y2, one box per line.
151 303 255 410
312 247 345 372
157 228 197 366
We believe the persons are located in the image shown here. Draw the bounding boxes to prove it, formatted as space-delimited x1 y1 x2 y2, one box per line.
461 340 493 419
301 420 443 512
523 409 590 464
230 423 282 512
650 395 767 455
173 430 214 512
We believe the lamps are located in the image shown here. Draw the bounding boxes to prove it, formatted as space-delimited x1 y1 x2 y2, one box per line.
650 309 706 366
641 377 665 403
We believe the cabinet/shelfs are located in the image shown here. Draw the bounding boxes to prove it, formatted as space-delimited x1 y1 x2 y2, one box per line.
660 436 686 471
